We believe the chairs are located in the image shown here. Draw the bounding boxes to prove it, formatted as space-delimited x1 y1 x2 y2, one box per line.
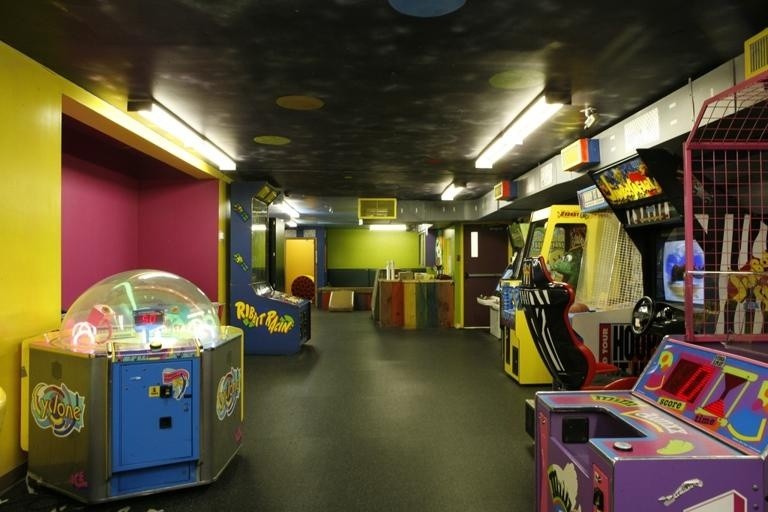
517 255 636 392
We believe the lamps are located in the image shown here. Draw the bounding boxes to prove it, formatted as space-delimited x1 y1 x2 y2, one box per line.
441 181 467 201
126 98 237 172
475 88 572 169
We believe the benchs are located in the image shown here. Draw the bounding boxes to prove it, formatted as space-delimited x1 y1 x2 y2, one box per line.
319 267 426 311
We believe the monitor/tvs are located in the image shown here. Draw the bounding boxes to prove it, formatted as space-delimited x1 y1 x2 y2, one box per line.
663 240 704 305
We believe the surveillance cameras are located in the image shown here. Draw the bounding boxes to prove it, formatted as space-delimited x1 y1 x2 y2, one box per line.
584 113 600 130
